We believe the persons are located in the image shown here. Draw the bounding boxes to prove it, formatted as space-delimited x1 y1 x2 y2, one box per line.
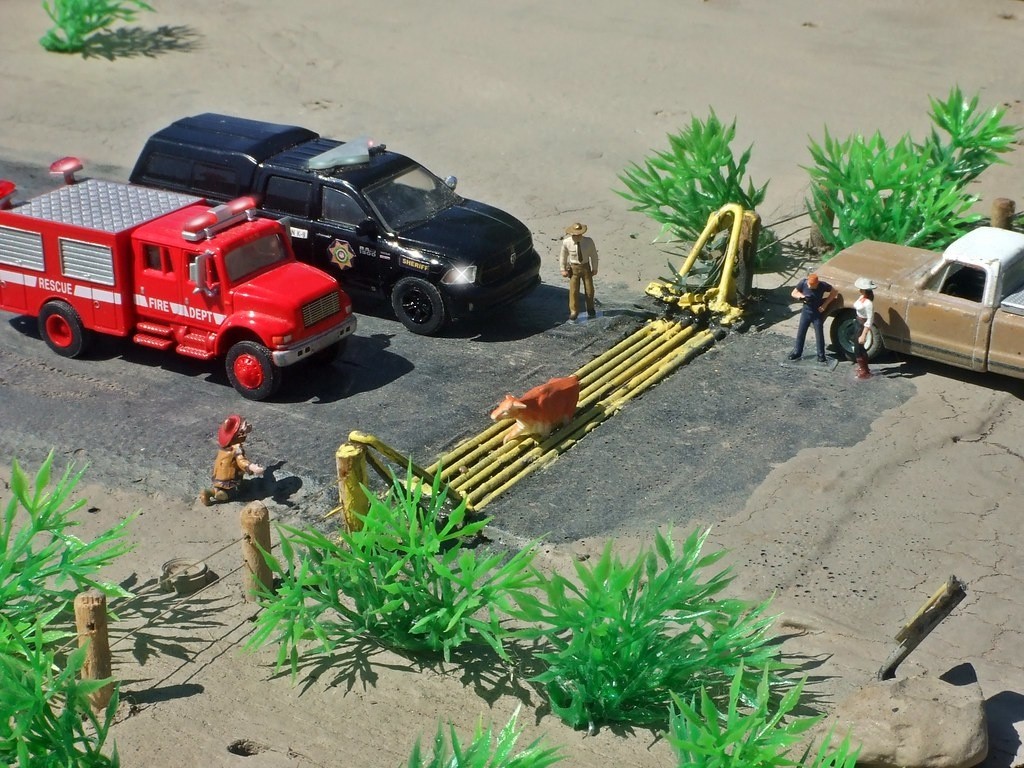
852 278 878 380
788 275 837 362
199 414 265 507
559 222 602 320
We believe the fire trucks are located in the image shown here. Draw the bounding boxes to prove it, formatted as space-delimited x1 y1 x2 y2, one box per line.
3 157 358 402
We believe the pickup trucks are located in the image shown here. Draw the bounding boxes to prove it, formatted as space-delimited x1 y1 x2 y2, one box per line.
823 223 1022 380
127 112 541 337
